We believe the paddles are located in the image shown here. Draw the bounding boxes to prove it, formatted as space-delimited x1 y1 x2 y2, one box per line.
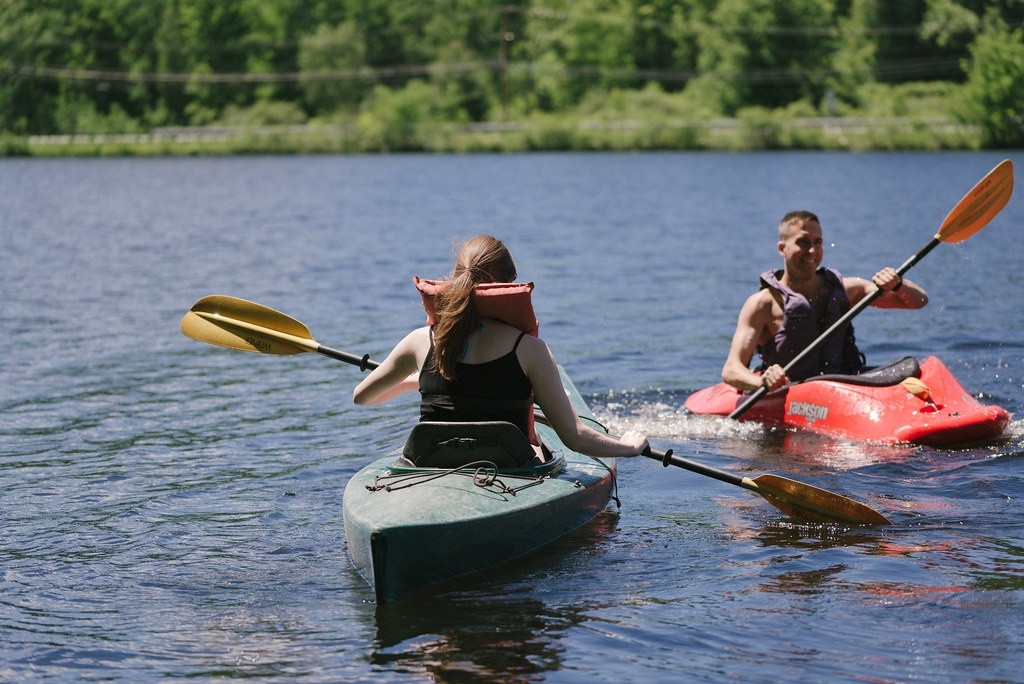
181 294 889 527
727 159 1013 421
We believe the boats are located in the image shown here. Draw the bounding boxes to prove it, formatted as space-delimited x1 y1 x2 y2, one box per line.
683 354 1008 449
341 358 618 603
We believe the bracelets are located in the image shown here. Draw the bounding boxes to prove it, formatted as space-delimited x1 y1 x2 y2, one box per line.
893 280 904 290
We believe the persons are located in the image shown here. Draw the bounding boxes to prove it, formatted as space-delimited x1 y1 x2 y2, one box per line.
353 235 649 457
719 211 928 388
